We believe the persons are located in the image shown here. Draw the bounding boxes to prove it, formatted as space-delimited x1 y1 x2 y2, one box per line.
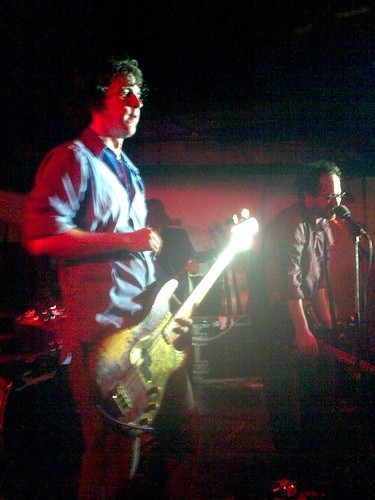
245 160 346 490
24 59 200 500
0 197 199 323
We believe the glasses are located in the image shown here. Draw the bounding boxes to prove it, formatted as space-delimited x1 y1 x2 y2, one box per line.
320 192 347 201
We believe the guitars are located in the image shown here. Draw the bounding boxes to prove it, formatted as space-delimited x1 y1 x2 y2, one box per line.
85 208 260 437
262 329 375 377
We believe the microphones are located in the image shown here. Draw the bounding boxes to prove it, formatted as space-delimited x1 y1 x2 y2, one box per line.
334 205 371 240
